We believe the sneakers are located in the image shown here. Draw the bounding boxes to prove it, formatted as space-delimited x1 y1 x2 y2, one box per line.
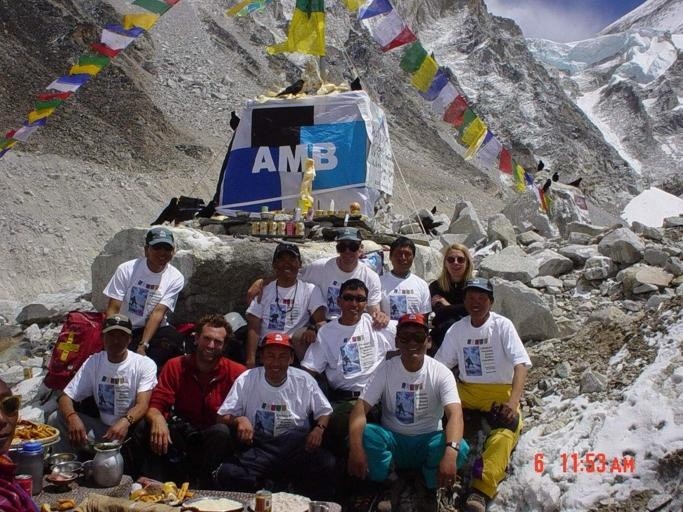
376 491 393 512
432 487 459 512
464 490 489 512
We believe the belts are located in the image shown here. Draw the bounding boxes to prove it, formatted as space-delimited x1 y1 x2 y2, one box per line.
329 389 362 400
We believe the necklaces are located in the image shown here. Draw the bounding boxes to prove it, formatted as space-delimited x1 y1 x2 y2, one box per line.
275 280 297 314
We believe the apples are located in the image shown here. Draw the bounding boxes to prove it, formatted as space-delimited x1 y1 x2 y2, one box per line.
163 482 178 496
350 202 360 211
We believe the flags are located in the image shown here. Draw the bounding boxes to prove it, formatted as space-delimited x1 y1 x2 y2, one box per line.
432 82 477 131
343 1 417 50
459 118 501 164
0 0 182 159
499 147 551 215
400 41 449 102
224 1 330 57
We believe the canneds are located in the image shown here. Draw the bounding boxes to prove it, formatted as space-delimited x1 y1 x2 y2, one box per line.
251 206 315 236
15 474 33 497
255 488 272 512
23 367 32 379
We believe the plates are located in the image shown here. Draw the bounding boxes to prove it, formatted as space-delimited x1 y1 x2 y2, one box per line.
247 491 313 511
179 497 244 511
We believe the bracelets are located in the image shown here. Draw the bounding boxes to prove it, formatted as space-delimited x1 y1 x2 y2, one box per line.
65 411 77 422
315 423 327 429
124 415 135 424
138 341 149 348
305 324 318 333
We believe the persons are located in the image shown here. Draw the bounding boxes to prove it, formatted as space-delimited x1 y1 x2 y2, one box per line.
215 328 335 496
241 238 327 369
121 313 249 492
243 224 388 331
297 278 390 438
379 235 431 322
100 226 184 378
0 377 42 511
344 312 472 512
427 244 476 352
44 312 159 474
342 202 368 227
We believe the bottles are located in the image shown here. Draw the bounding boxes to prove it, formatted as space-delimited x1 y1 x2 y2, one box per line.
90 439 126 487
250 206 314 236
18 441 44 495
256 489 273 512
24 368 33 380
488 399 520 433
313 210 351 219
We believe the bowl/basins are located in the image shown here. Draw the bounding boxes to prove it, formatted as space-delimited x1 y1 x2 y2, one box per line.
47 450 95 489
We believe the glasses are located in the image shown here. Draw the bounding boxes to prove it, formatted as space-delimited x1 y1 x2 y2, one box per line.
337 292 370 304
0 393 25 418
335 241 363 253
444 254 467 265
396 330 427 344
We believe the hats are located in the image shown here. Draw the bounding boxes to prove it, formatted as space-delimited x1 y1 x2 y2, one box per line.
463 276 495 295
145 227 176 250
397 311 429 330
333 226 364 244
259 332 294 351
271 241 302 260
100 313 133 336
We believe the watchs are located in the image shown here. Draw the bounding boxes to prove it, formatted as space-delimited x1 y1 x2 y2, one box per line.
445 440 459 451
